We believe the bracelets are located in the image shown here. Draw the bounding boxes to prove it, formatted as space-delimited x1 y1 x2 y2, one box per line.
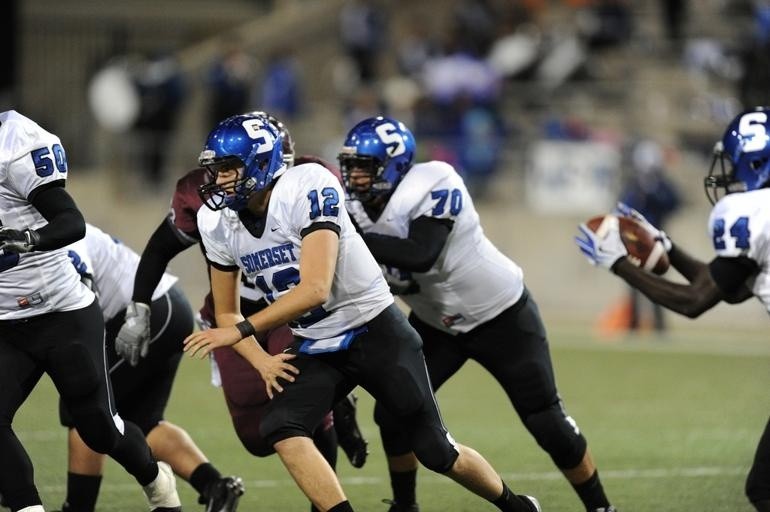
235 320 256 339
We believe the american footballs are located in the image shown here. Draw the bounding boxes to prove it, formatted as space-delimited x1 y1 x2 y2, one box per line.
585 216 670 276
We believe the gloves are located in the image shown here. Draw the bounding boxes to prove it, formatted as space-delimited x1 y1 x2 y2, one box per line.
115 300 151 367
379 264 421 295
0 227 40 252
575 216 628 270
610 201 672 253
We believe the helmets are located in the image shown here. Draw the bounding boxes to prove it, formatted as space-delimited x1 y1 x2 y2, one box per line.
704 105 770 206
336 115 416 208
197 111 296 210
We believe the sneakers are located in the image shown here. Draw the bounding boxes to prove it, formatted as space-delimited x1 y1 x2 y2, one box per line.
199 476 245 512
142 461 182 512
502 494 541 512
382 499 420 512
333 393 369 468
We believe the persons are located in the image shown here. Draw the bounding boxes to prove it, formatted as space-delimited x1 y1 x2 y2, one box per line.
58 222 244 512
336 117 617 512
115 146 370 512
0 109 181 512
575 105 770 512
182 112 541 512
